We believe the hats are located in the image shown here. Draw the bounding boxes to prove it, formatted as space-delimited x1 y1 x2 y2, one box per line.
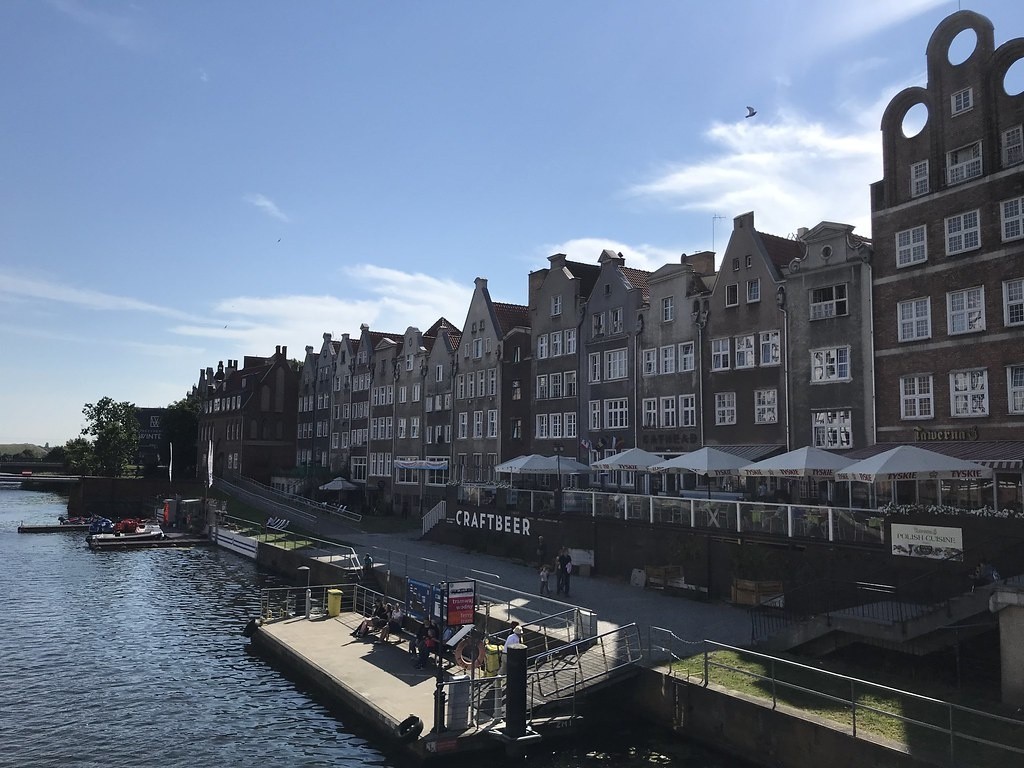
513 626 523 633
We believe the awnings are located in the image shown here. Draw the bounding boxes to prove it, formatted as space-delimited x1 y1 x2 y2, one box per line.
682 441 1024 470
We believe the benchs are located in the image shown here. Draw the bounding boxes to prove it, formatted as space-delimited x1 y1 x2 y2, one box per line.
371 616 440 667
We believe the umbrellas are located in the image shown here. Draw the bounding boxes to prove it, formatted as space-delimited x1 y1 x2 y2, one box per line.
319 477 358 503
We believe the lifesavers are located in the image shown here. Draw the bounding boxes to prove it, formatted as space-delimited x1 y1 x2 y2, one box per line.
454 639 486 669
187 515 191 525
392 715 424 743
242 619 260 638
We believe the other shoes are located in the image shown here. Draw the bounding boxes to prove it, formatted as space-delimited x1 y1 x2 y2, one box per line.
417 667 424 670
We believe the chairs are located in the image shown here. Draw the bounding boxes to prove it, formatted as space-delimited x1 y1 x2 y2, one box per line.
580 493 867 542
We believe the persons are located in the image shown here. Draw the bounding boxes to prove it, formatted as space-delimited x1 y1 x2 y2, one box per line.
266 514 274 528
409 619 439 671
757 481 766 499
493 621 523 687
764 498 785 527
540 546 571 600
350 599 403 644
401 499 408 520
819 500 833 536
363 553 372 575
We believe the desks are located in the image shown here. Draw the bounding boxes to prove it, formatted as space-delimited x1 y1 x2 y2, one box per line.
586 497 603 514
801 511 826 529
661 503 681 523
751 509 777 527
627 501 641 517
704 506 727 528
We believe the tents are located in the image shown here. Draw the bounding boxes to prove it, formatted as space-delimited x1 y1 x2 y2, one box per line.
835 445 997 510
590 447 665 494
494 454 591 490
647 447 755 500
738 446 858 501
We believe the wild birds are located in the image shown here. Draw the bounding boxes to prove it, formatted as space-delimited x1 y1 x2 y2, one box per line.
745 107 758 119
276 238 281 243
224 324 227 329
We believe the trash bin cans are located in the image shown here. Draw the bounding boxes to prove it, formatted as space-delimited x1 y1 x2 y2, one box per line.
484 644 503 673
327 589 344 617
